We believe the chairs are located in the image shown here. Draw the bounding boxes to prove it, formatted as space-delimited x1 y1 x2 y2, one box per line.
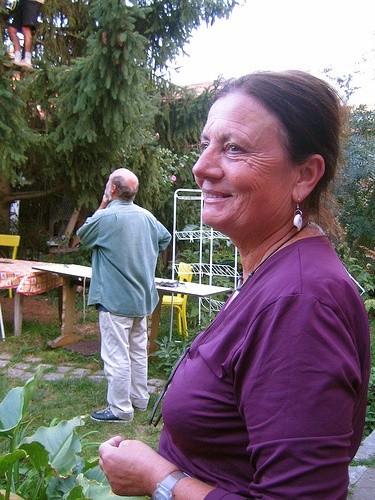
0 234 21 298
161 261 194 338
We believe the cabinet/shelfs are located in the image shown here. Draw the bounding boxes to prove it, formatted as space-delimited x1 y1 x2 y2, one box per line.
168 186 240 349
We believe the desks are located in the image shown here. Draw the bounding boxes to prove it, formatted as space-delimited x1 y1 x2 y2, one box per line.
0 257 64 336
33 263 231 359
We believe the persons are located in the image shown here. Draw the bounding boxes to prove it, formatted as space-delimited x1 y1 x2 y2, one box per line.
75 168 171 421
96 69 372 500
4 0 44 69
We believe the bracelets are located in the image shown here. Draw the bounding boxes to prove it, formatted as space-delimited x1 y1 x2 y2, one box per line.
151 470 191 500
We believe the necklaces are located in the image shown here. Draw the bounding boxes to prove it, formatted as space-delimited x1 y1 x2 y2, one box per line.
235 219 309 291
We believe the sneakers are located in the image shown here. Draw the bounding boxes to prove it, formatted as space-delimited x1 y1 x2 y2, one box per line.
90 400 148 423
